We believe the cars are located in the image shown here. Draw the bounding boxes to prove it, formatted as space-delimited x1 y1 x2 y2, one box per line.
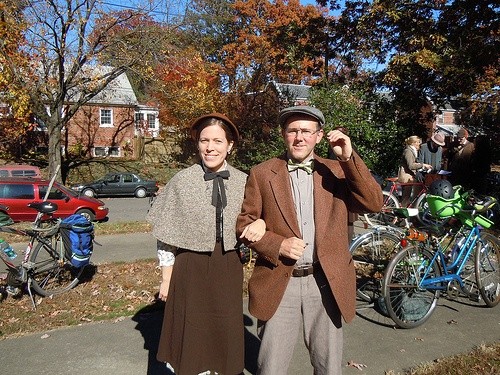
0 176 110 226
68 172 159 199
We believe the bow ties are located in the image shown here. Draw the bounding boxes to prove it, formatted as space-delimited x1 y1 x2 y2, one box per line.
204 170 231 208
287 158 314 174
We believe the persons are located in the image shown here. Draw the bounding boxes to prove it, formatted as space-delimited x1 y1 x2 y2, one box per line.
398 127 476 228
145 114 266 375
236 105 383 375
63 156 69 186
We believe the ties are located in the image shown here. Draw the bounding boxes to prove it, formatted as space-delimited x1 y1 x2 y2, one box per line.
431 148 437 169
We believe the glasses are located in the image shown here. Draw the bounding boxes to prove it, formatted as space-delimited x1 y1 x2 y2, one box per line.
281 129 320 137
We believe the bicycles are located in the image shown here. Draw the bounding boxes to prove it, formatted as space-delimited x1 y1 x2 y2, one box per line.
0 201 94 309
348 167 500 329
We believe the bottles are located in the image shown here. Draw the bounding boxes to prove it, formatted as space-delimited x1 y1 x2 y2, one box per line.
405 229 426 241
0 239 17 261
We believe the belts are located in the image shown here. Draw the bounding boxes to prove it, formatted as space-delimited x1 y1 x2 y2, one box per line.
291 265 322 277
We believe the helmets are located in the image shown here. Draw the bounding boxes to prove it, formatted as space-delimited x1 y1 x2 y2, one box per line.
431 179 454 199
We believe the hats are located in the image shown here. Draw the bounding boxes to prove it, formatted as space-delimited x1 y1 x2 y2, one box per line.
431 134 445 146
279 106 326 128
191 112 240 145
457 128 469 138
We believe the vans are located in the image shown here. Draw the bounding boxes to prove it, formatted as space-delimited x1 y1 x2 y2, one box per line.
0 164 44 179
